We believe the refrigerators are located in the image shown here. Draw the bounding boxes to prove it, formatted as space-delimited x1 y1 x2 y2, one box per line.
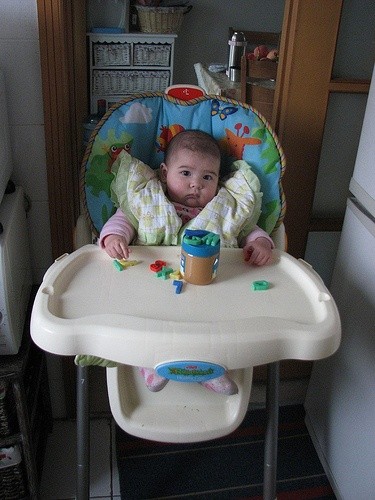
302 65 375 499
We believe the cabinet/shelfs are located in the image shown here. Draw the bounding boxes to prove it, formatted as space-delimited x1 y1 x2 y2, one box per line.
87 32 178 118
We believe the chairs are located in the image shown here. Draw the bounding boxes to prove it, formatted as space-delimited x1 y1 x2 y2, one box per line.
79 84 287 444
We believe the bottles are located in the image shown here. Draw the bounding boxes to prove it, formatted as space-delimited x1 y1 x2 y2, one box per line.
225 32 247 76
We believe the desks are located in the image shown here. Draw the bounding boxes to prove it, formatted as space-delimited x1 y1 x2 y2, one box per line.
0 277 52 500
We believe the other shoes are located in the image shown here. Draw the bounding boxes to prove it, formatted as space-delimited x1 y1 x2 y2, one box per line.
200 373 239 395
140 367 169 392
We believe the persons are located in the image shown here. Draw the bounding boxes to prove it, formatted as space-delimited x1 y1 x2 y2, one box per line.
98 127 276 397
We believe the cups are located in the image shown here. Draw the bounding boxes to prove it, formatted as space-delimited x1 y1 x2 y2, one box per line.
230 66 242 81
